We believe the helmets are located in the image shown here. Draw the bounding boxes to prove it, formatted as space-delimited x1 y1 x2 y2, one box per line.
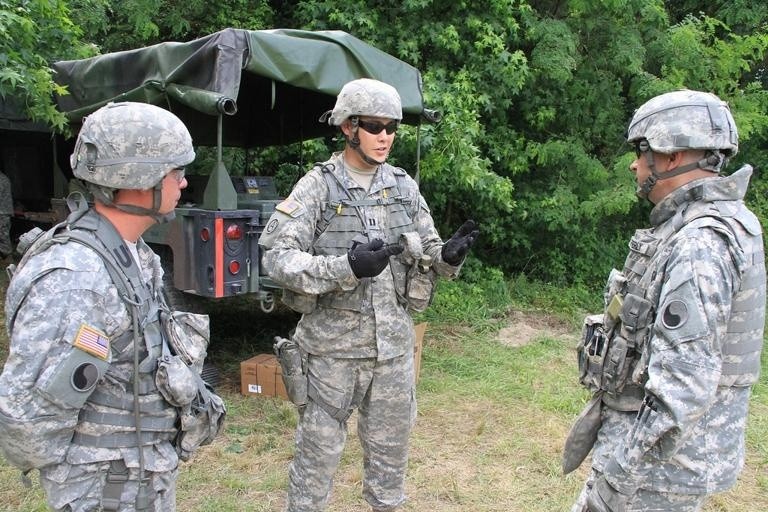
70 101 197 191
627 89 738 157
330 77 405 126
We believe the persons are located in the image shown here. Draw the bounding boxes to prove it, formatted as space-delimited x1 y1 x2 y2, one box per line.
1 101 227 512
255 77 481 511
562 88 768 510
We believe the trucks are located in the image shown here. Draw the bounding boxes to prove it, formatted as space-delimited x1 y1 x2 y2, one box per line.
0 27 422 314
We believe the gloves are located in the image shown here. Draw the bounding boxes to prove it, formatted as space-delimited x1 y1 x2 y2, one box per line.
348 237 403 279
441 220 479 267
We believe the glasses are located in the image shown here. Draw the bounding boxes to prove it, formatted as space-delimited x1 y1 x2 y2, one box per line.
171 168 185 182
358 118 399 135
